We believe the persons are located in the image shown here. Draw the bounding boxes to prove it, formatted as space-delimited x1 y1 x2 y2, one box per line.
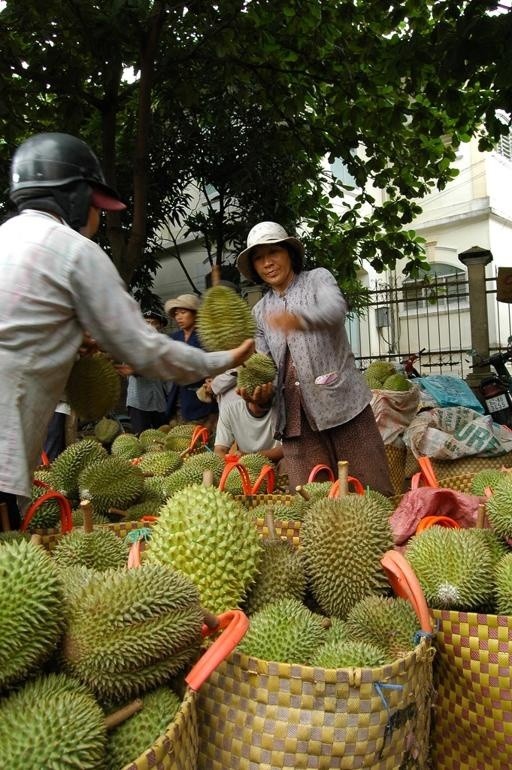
42 396 74 462
0 129 256 534
127 306 170 433
158 293 220 427
233 220 397 498
212 381 286 471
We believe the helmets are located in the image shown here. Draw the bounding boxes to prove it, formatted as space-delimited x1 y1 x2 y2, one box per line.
9 132 126 225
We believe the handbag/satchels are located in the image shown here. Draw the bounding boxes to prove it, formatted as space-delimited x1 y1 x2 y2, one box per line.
409 516 509 768
127 534 434 768
122 610 249 768
20 426 508 578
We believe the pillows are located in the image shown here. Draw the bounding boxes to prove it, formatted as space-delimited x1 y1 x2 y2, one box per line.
9 132 126 225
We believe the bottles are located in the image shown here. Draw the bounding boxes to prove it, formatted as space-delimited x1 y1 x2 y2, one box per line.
165 295 199 315
238 221 305 282
143 310 167 327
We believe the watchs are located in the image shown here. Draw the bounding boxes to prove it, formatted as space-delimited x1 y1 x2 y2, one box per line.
398 349 426 380
466 334 512 429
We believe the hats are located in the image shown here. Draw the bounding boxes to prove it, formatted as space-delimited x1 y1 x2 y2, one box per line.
238 221 305 282
165 295 199 315
143 310 167 327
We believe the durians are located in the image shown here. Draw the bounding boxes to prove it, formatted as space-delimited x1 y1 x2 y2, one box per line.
0 416 512 770
66 355 120 417
363 361 413 392
237 352 276 398
195 287 258 353
